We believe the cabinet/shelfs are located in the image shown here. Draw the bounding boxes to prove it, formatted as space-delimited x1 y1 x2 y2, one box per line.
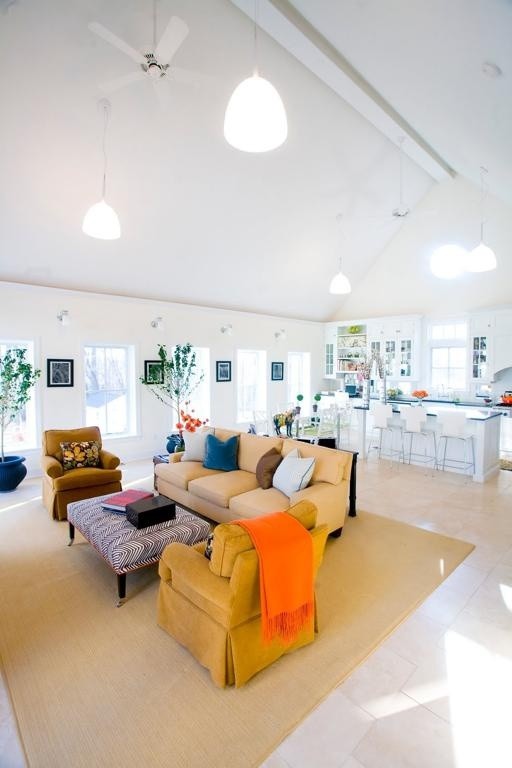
323 322 344 380
335 320 368 373
468 308 512 384
368 314 427 381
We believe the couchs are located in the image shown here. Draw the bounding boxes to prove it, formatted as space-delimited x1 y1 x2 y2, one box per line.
158 501 329 689
156 426 353 537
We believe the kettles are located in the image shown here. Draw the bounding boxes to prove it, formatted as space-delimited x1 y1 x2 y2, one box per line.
501 390 512 403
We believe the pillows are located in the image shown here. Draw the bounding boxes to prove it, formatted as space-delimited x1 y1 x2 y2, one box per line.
203 434 239 472
60 441 101 471
181 428 215 461
256 448 283 489
272 448 315 498
205 532 214 559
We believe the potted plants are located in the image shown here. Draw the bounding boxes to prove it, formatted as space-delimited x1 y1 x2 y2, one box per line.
139 343 205 453
0 349 41 490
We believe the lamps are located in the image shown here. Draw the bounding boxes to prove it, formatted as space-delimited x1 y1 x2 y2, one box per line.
392 134 409 217
82 99 121 241
329 255 351 294
471 219 496 272
224 1 289 153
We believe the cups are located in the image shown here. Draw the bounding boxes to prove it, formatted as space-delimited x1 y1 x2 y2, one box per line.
484 398 493 403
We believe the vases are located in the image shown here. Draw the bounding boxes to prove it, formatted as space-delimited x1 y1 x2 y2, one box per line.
175 441 186 452
417 397 422 406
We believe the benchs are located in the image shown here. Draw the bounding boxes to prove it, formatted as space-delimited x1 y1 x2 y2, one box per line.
66 486 210 608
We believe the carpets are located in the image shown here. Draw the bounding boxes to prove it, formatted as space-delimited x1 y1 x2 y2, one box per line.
0 477 476 767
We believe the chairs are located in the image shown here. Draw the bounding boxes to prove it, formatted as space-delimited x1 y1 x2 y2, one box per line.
322 397 352 448
367 405 404 463
398 407 437 466
433 411 476 477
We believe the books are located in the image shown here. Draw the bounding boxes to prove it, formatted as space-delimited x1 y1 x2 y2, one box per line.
99 489 154 512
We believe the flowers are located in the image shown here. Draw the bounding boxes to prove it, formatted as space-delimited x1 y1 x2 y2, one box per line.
413 390 428 398
177 410 209 438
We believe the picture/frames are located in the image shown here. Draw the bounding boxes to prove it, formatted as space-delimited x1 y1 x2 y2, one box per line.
216 361 231 382
47 359 74 387
271 362 283 380
144 360 165 384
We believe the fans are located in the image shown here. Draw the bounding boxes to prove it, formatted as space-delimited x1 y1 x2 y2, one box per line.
88 0 215 111
